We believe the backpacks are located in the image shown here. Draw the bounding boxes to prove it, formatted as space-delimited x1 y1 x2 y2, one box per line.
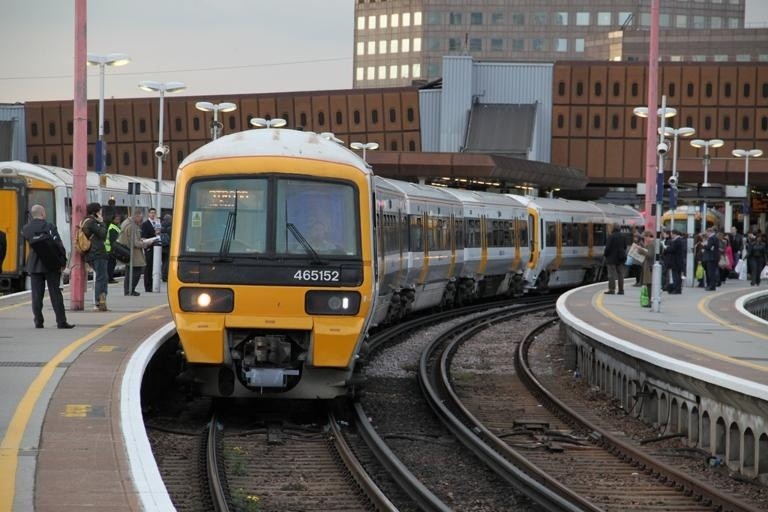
74 218 91 254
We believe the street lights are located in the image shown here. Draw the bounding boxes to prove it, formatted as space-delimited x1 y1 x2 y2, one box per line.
84 51 133 211
631 104 678 122
657 125 697 236
249 117 288 129
193 101 239 141
688 138 724 231
350 141 379 161
136 78 186 293
729 148 764 241
317 130 336 141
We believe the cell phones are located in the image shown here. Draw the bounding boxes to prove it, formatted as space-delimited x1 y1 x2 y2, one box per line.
94 213 98 218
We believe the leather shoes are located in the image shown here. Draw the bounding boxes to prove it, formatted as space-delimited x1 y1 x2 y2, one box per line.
58 323 75 328
36 324 43 328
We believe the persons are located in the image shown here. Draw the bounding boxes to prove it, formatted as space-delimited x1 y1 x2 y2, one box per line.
104 213 121 283
632 225 768 295
82 202 108 312
120 207 174 296
604 223 627 294
22 204 75 329
297 222 337 252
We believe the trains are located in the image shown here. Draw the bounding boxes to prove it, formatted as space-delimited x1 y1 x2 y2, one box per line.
163 127 649 417
0 159 174 291
660 206 725 237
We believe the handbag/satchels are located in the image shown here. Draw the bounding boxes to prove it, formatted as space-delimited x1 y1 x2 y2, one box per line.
759 265 768 279
29 223 66 270
109 240 131 264
735 260 743 273
696 265 704 279
